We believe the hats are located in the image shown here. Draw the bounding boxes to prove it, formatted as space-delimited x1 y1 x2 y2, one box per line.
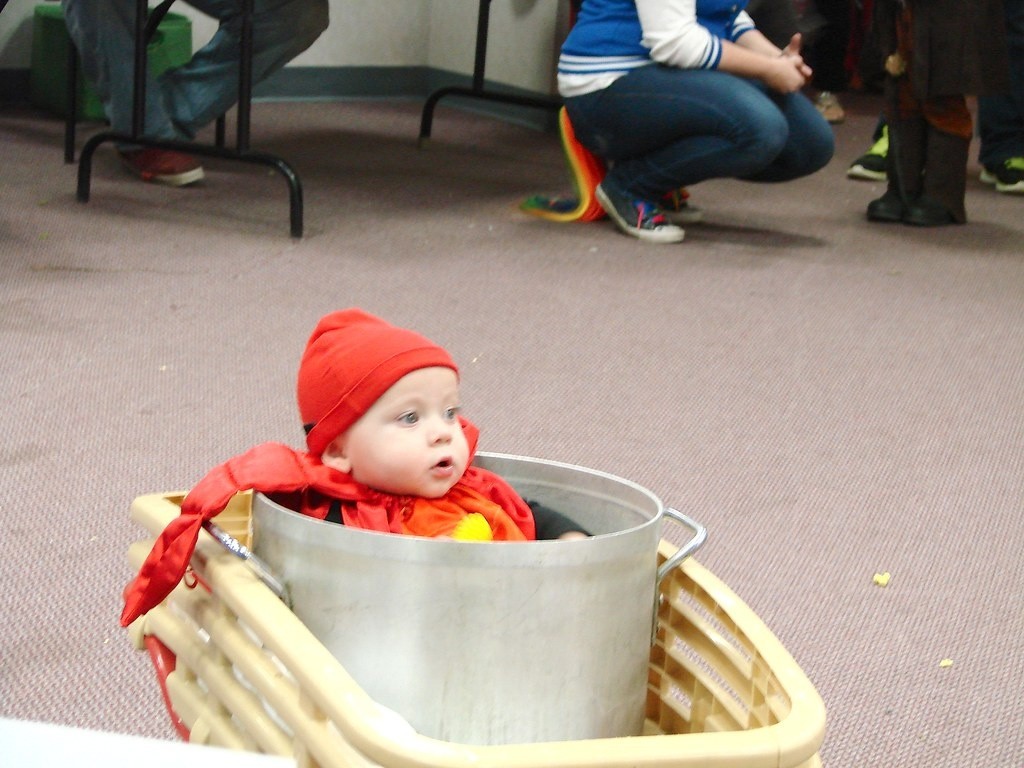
295 307 460 457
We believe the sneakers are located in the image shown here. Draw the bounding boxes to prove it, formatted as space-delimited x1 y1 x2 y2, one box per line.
663 188 703 223
595 170 686 243
980 155 1024 193
846 111 890 181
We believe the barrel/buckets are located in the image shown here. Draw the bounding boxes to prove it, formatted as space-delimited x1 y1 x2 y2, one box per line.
250 451 708 741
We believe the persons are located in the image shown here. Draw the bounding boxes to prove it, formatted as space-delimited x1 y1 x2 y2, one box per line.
61 0 329 188
747 1 1024 226
557 0 833 241
296 310 594 541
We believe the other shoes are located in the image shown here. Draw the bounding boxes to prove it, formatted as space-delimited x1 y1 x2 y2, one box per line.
814 94 845 124
119 143 205 186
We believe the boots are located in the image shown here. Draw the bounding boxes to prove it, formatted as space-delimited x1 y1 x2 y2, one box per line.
903 127 970 225
866 125 926 221
